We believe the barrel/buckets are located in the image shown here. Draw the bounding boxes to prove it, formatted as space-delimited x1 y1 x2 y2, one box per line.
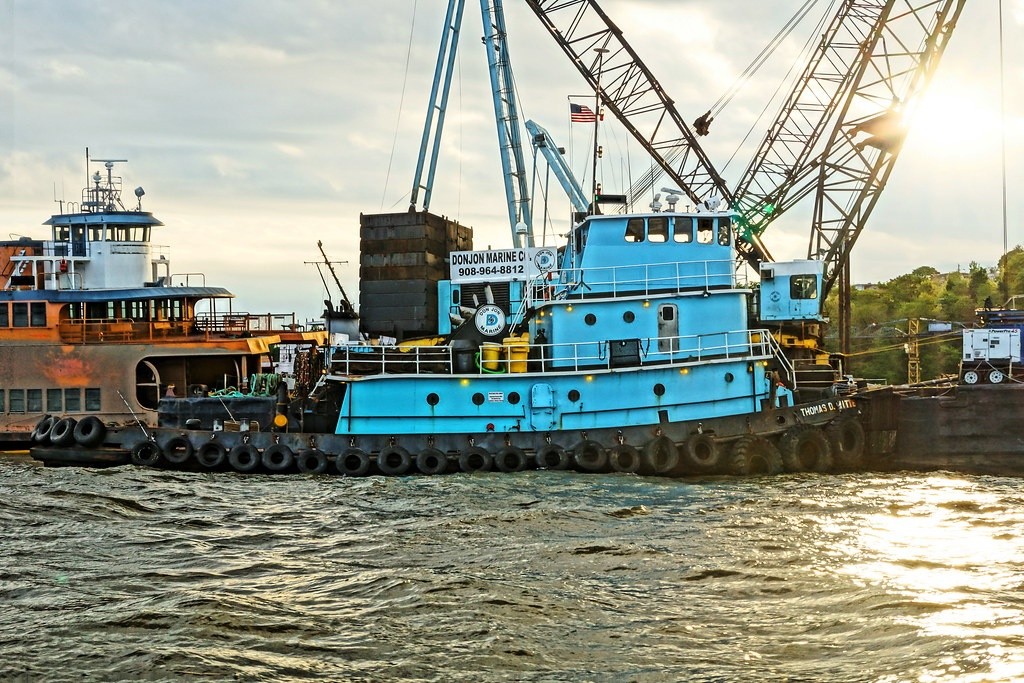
482 344 502 370
455 348 474 372
503 337 530 373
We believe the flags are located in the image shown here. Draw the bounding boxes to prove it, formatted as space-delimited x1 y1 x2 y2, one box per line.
571 103 596 122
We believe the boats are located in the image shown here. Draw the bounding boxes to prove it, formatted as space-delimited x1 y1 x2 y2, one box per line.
1 159 281 454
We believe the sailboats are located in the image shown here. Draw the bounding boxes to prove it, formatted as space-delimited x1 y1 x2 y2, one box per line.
30 0 899 478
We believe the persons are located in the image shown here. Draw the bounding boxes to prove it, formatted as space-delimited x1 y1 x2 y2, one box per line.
769 368 783 410
